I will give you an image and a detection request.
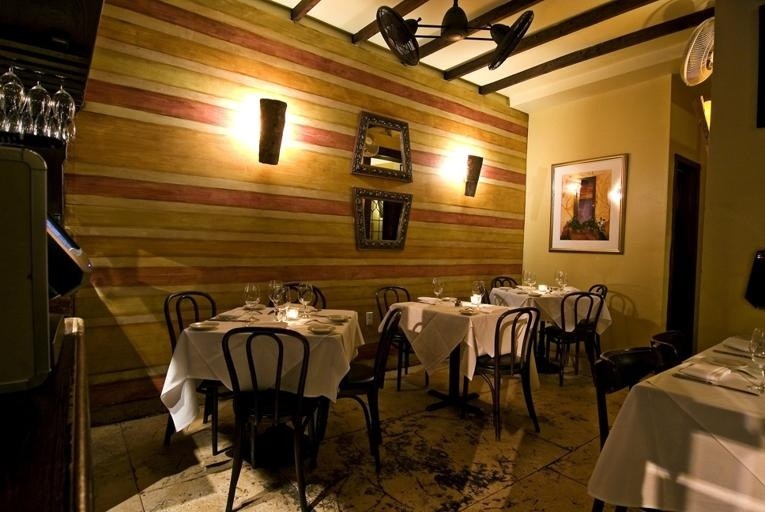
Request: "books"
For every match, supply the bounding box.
[672,360,765,395]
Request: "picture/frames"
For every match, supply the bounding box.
[548,152,630,256]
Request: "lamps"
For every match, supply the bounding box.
[464,154,484,197]
[258,98,288,166]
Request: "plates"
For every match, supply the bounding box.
[243,304,265,309]
[219,314,242,319]
[309,324,334,336]
[460,312,477,316]
[328,315,350,323]
[190,322,220,330]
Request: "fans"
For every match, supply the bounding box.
[680,16,715,86]
[376,0,534,71]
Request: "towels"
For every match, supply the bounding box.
[679,362,732,383]
[721,336,760,353]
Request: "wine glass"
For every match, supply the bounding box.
[472,280,486,309]
[523,271,527,292]
[432,277,444,300]
[268,281,280,316]
[276,287,290,321]
[559,271,567,292]
[555,271,561,287]
[750,327,765,389]
[1,68,78,139]
[298,280,313,317]
[526,272,536,292]
[242,282,260,322]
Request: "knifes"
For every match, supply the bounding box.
[672,372,759,396]
[712,349,753,359]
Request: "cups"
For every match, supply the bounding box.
[470,296,481,305]
[538,284,547,292]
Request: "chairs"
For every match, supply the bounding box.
[163,277,687,511]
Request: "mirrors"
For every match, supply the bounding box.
[351,186,413,250]
[350,110,414,183]
